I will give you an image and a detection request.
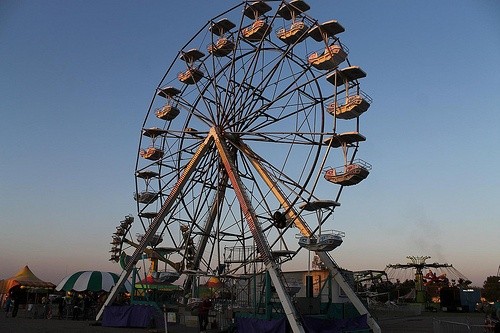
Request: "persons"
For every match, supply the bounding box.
[9,283,23,317]
[41,292,90,322]
[198,296,212,333]
[6,295,11,313]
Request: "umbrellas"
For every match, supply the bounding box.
[54,271,136,294]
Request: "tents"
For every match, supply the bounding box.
[135,271,181,304]
[0,264,57,305]
